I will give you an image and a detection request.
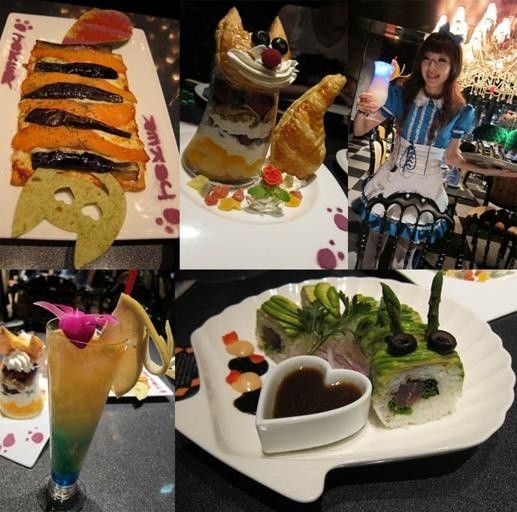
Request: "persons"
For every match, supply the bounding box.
[352,33,517,270]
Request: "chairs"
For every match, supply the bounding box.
[360,124,517,270]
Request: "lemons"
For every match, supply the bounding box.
[98,292,175,399]
[390,59,411,82]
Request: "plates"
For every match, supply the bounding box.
[179,121,348,269]
[0,12,179,241]
[0,364,174,468]
[174,270,516,503]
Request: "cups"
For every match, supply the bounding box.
[36,312,129,511]
[181,72,278,186]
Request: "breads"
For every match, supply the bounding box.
[272,72,347,178]
[257,272,465,427]
[215,6,300,95]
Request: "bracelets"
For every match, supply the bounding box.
[356,110,370,117]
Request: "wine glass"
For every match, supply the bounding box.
[362,61,393,123]
